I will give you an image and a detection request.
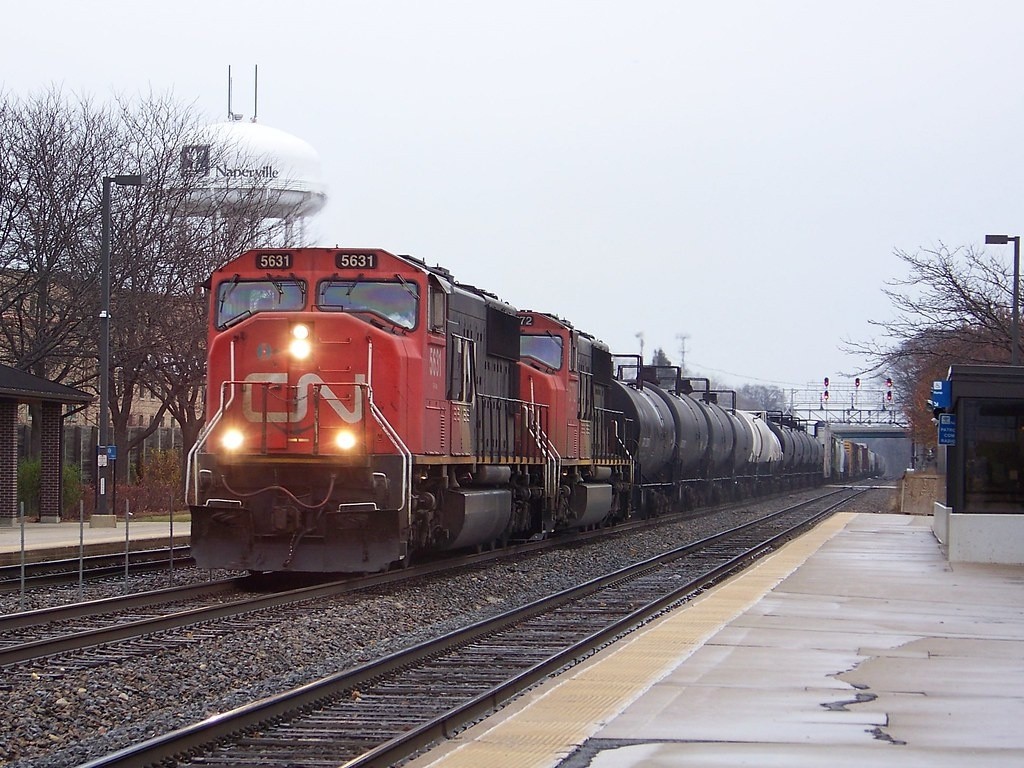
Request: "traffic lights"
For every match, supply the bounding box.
[823,377,892,401]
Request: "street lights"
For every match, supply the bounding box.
[97,175,149,514]
[986,236,1018,365]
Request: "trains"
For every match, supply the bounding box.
[181,246,887,575]
